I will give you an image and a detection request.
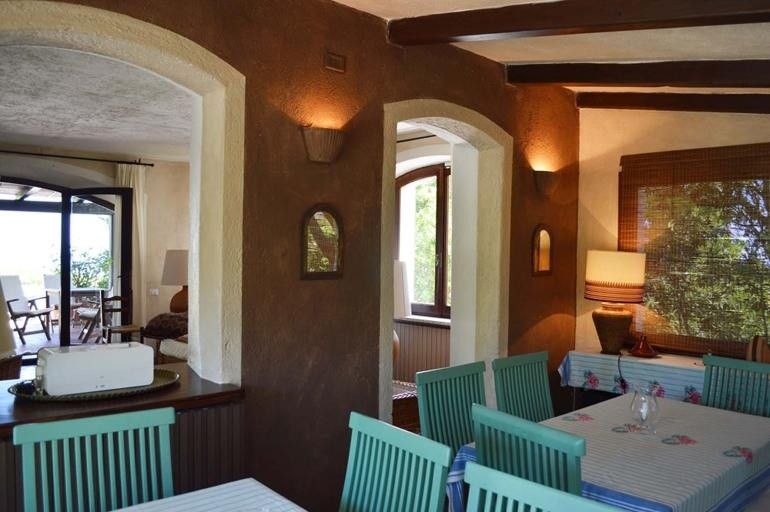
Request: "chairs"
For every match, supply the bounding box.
[100,291,145,347]
[337,411,453,512]
[1,271,114,346]
[13,406,175,512]
[417,346,770,512]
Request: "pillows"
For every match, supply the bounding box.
[146,312,191,342]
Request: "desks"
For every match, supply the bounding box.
[119,476,305,511]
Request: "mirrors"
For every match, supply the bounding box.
[302,203,346,281]
[531,223,555,278]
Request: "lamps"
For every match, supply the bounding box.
[535,169,561,197]
[303,123,349,168]
[582,248,649,358]
[161,249,189,314]
[389,258,415,373]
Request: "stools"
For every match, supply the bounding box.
[160,335,189,366]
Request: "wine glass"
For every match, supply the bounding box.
[629,382,661,436]
